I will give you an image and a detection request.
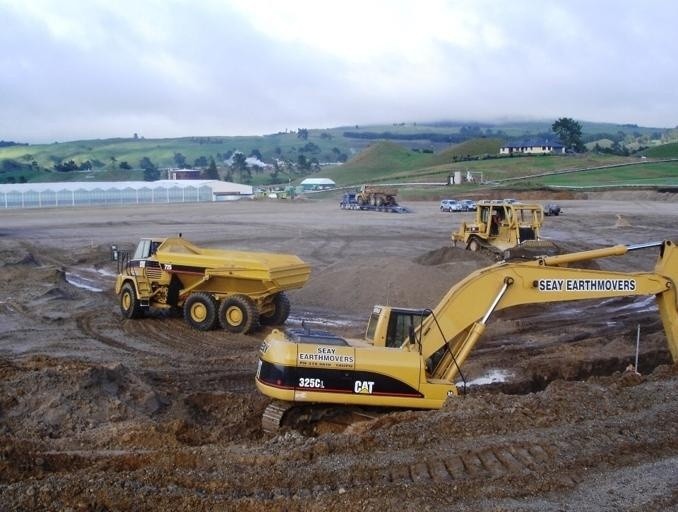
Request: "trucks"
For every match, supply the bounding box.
[107,234,316,336]
[353,183,399,207]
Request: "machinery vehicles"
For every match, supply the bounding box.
[450,203,561,262]
[249,236,678,439]
[338,191,414,214]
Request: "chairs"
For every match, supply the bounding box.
[491,216,497,235]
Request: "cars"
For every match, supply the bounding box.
[439,196,562,217]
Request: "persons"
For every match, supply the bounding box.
[492,208,503,234]
